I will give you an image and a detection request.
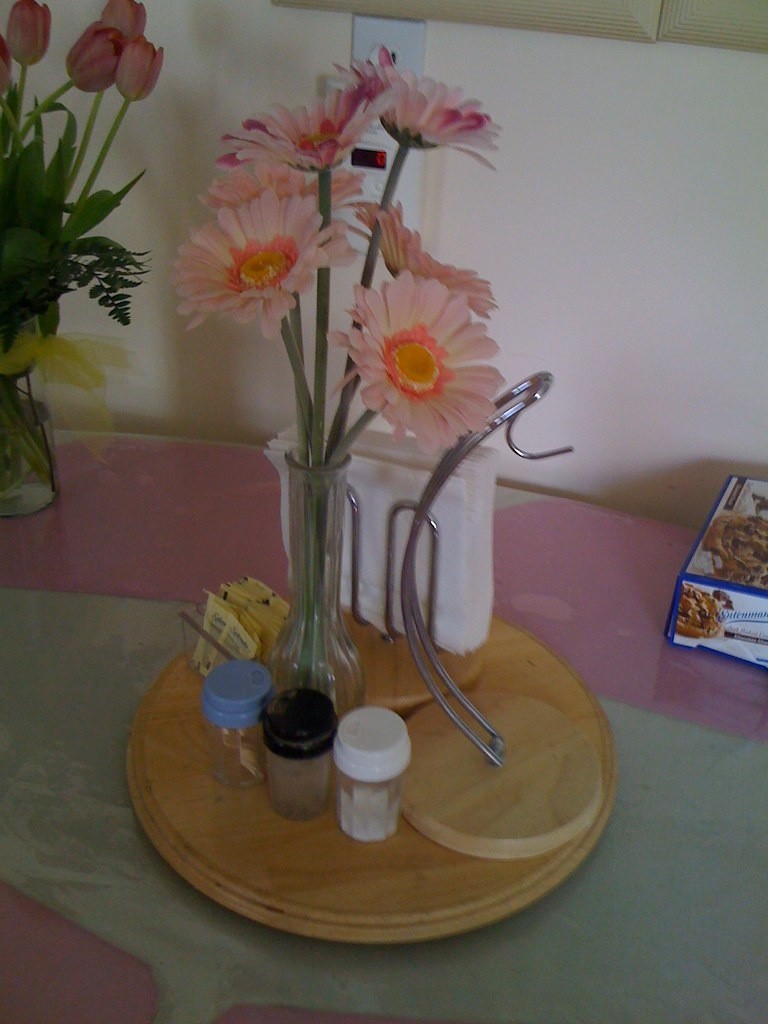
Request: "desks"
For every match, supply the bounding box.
[0,428,768,1024]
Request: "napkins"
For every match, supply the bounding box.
[264,420,498,658]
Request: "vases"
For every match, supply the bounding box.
[263,448,365,727]
[0,318,59,520]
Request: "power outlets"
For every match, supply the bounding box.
[350,13,426,79]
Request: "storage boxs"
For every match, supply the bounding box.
[661,474,767,669]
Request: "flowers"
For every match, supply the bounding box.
[172,34,505,696]
[0,0,165,487]
